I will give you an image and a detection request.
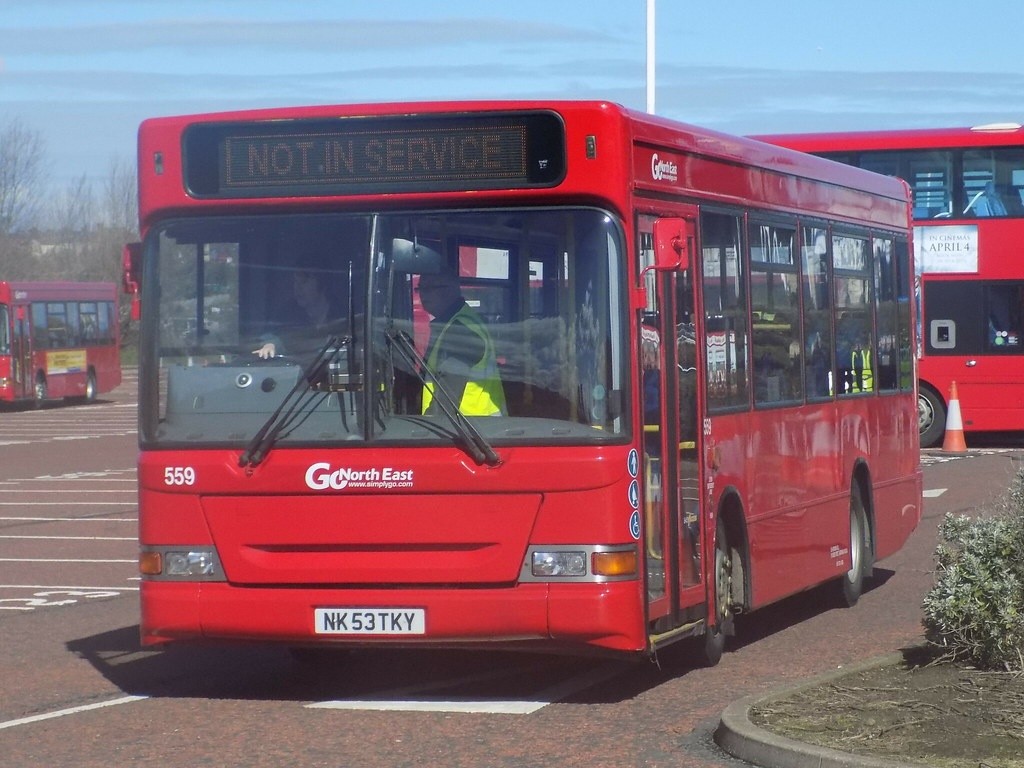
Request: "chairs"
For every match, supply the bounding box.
[944,181,1024,216]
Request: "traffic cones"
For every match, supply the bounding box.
[928,375,981,459]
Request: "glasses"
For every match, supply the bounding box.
[414,284,449,295]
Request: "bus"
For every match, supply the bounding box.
[121,100,925,671]
[410,122,1024,450]
[1,280,124,411]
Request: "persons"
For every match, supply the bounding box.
[411,263,505,420]
[848,337,874,394]
[246,257,363,363]
